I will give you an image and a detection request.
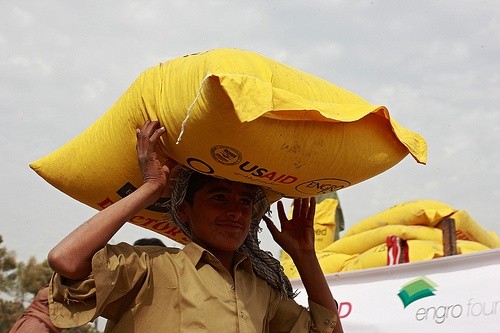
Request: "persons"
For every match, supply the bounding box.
[48,119,344,332]
[8,285,63,333]
